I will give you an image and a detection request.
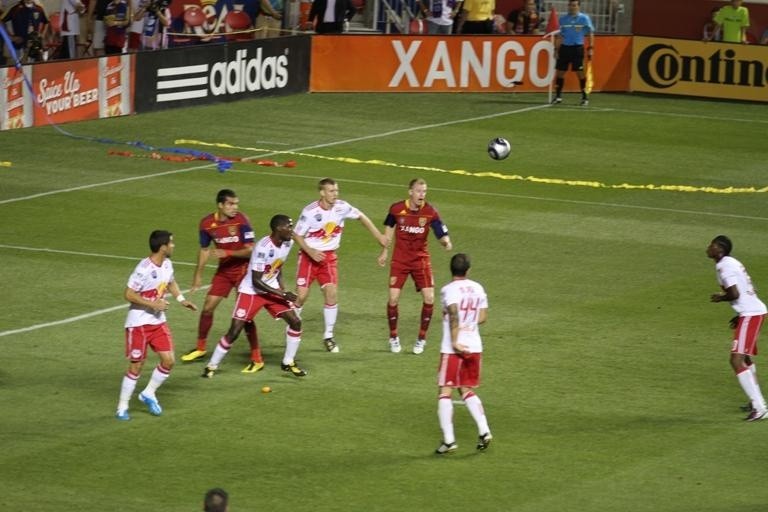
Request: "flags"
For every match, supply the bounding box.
[583,60,594,96]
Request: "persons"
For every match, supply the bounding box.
[180,189,264,373]
[552,0,596,106]
[116,230,198,421]
[286,179,392,352]
[201,214,307,377]
[377,179,452,354]
[1,0,357,70]
[379,0,547,34]
[706,236,768,422]
[204,488,228,512]
[702,0,768,45]
[436,254,493,454]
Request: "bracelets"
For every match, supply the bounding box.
[587,46,594,51]
[225,249,232,256]
[176,294,185,302]
[283,291,287,297]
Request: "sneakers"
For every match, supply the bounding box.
[551,97,561,104]
[477,433,492,451]
[580,99,589,106]
[181,336,425,380]
[138,392,162,416]
[115,409,130,421]
[739,402,763,422]
[435,439,458,455]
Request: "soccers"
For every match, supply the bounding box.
[488,138,510,160]
